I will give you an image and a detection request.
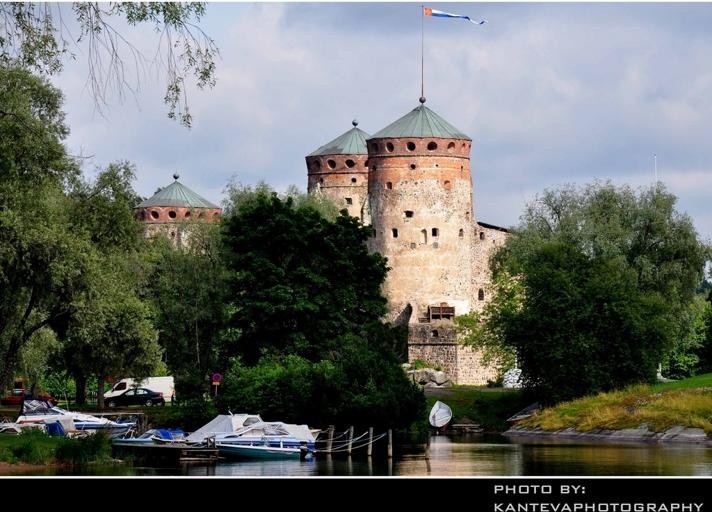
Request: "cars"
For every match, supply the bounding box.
[104,388,163,407]
[0,388,57,407]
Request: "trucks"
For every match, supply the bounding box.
[103,376,175,406]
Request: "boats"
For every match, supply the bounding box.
[506,401,540,424]
[429,399,453,428]
[0,412,322,461]
